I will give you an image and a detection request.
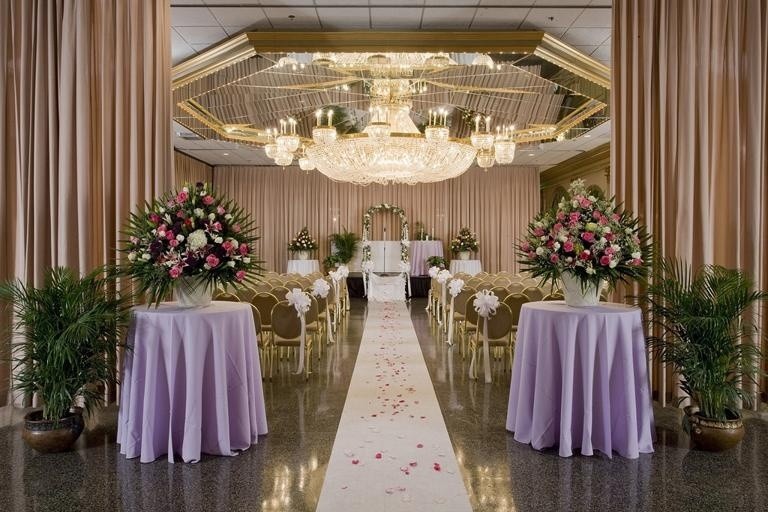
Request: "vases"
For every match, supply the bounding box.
[559,268,606,307]
[459,249,471,261]
[169,268,212,309]
[298,248,309,260]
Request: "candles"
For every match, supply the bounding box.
[419,224,436,241]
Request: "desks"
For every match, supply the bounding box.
[522,300,642,459]
[288,259,319,276]
[130,301,250,462]
[450,260,481,276]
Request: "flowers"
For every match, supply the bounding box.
[111,179,268,310]
[450,226,480,254]
[287,226,318,250]
[511,175,657,297]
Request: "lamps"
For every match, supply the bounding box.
[268,50,497,101]
[260,105,519,189]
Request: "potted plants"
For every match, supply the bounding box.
[0,264,142,454]
[623,254,768,452]
[322,225,359,274]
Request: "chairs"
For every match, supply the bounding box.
[423,266,563,383]
[211,271,349,384]
